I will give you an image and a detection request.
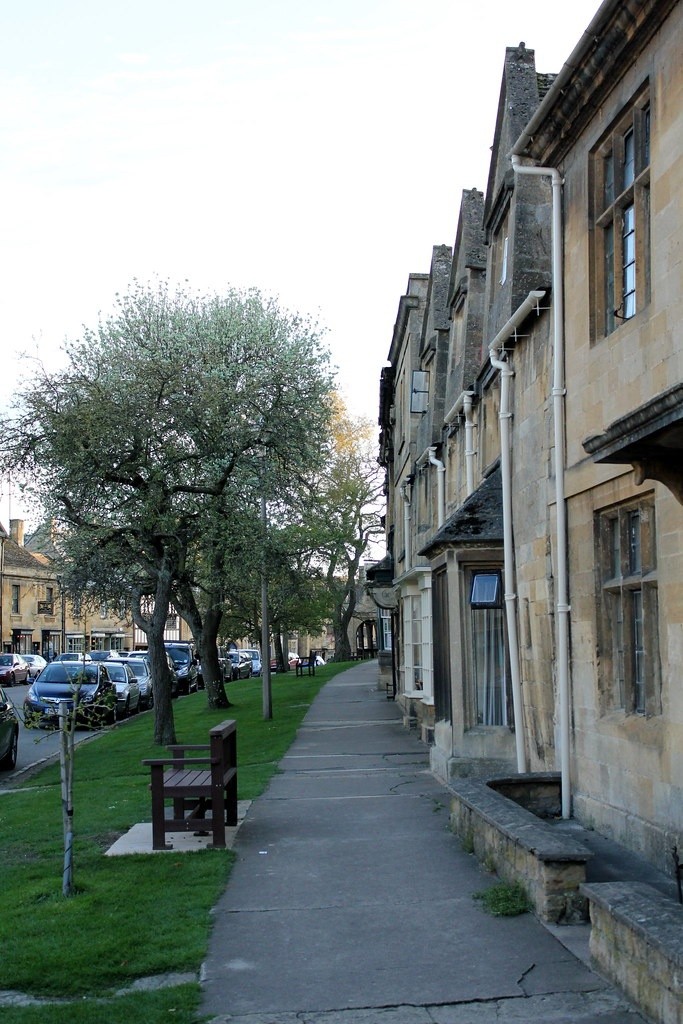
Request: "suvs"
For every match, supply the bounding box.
[87,649,122,660]
[161,643,199,695]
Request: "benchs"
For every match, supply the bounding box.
[350,650,364,661]
[140,719,237,851]
[295,650,316,677]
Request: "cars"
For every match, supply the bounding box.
[269,652,301,671]
[23,662,118,724]
[127,650,150,661]
[54,652,92,662]
[0,685,20,772]
[108,657,154,710]
[225,647,262,680]
[194,646,233,688]
[302,656,328,669]
[116,651,131,659]
[99,661,142,717]
[0,653,31,688]
[19,654,48,680]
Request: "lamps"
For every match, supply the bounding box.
[469,571,505,610]
